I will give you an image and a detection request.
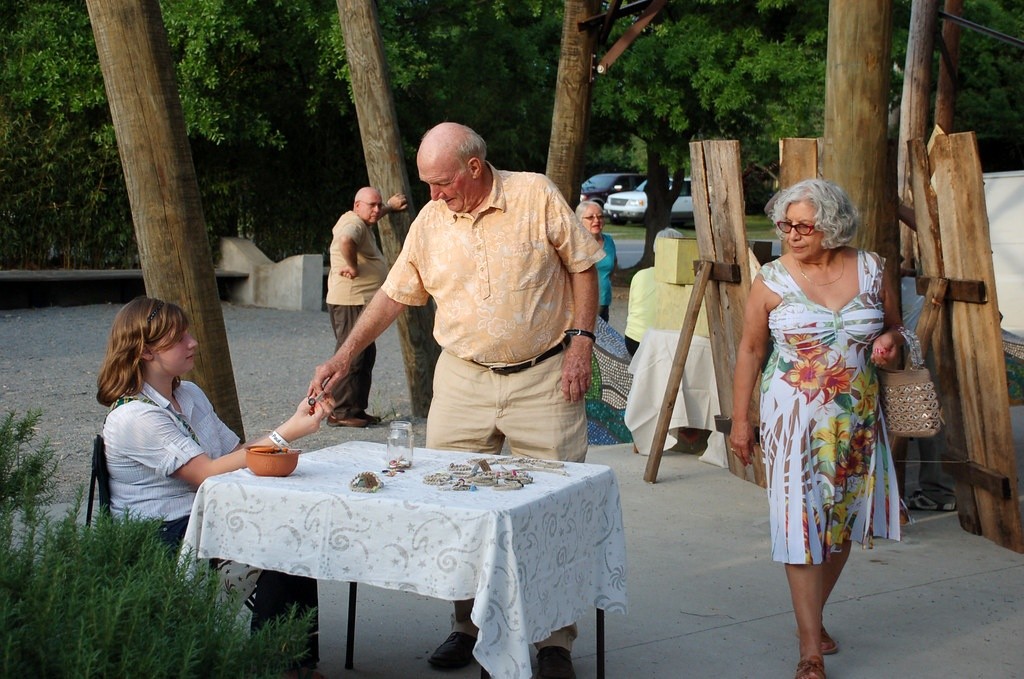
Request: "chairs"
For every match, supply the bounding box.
[84,431,306,634]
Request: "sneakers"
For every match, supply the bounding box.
[904,488,956,511]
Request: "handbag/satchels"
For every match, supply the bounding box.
[878,326,946,438]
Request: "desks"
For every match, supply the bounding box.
[176,440,627,679]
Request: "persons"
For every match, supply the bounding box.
[575,201,684,356]
[97,296,337,679]
[326,187,409,426]
[730,177,908,679]
[307,122,605,679]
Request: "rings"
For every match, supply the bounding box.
[731,447,734,451]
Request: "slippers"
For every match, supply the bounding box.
[796,624,838,679]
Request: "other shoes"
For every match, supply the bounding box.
[283,669,327,679]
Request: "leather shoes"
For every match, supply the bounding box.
[326,412,381,428]
[536,646,575,679]
[427,632,477,670]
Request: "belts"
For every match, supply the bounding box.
[470,335,571,376]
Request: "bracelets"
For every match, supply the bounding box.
[268,430,288,447]
[564,329,596,341]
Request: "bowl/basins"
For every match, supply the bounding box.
[243,445,302,477]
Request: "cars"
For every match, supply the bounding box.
[580,173,647,210]
[603,178,693,225]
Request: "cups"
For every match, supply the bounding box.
[387,421,414,469]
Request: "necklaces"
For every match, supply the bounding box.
[798,248,845,287]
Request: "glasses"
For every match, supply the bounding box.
[777,221,814,236]
[360,200,385,208]
[583,215,605,220]
